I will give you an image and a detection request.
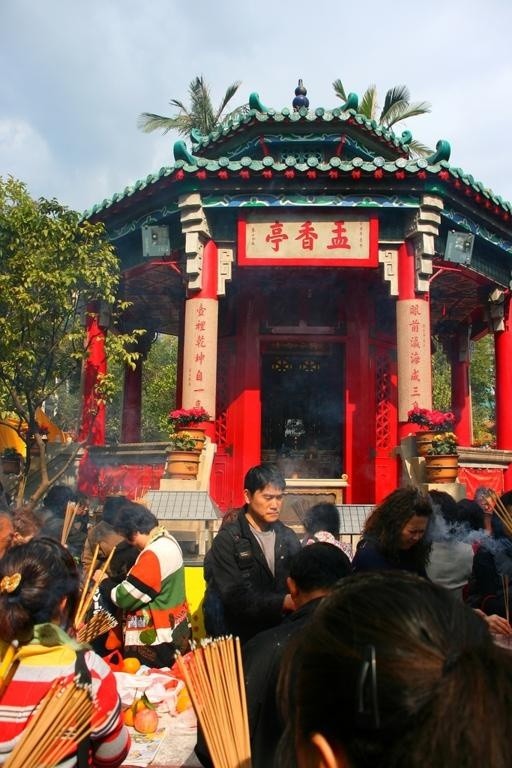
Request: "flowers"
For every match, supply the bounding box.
[169,407,210,425]
[428,432,457,455]
[407,407,455,432]
[168,429,195,451]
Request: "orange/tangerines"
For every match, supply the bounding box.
[123,658,140,674]
[135,701,147,713]
[125,709,134,726]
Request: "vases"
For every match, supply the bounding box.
[166,449,202,480]
[170,428,205,450]
[424,456,460,483]
[416,432,435,456]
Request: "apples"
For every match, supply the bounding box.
[134,709,158,733]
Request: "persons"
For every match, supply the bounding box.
[102,496,145,585]
[196,543,352,767]
[93,502,192,668]
[424,490,474,602]
[474,491,511,620]
[81,521,127,592]
[299,502,359,561]
[0,535,130,768]
[31,486,87,574]
[0,509,17,560]
[353,486,512,638]
[9,513,43,547]
[276,568,512,768]
[458,498,493,619]
[211,463,302,646]
